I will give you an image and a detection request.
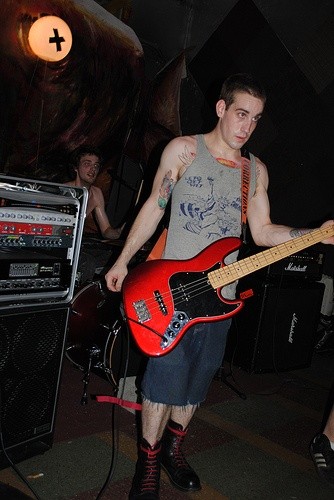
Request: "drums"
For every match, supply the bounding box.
[64,280,149,389]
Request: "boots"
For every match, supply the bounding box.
[128,437,163,500]
[158,418,202,492]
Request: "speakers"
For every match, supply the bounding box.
[0,306,70,470]
[225,275,325,375]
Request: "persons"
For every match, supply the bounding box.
[310,401,334,489]
[105,74,334,500]
[60,145,122,276]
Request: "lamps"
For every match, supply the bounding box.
[29,16,74,62]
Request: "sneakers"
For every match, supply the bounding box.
[311,434,334,486]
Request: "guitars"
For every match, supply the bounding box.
[121,224,334,358]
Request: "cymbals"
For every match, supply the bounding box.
[106,238,155,252]
